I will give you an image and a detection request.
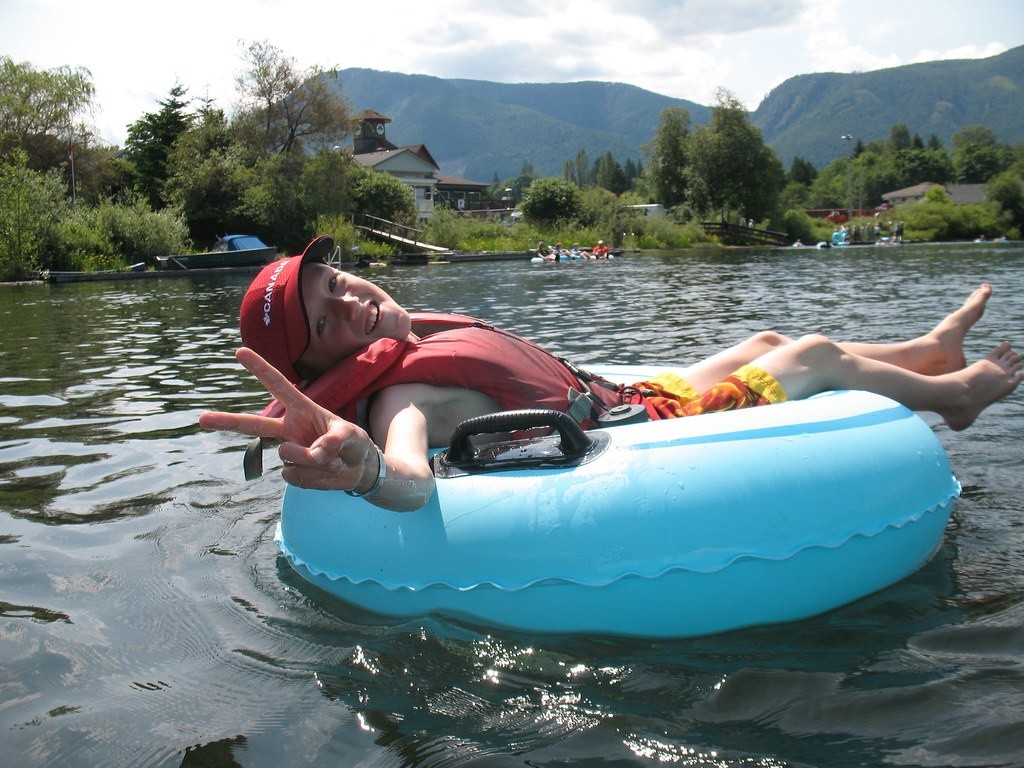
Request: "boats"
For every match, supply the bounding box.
[355,258,388,267]
[154,233,278,268]
[39,263,146,282]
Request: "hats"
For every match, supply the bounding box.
[749,219,754,222]
[598,240,603,244]
[240,234,334,388]
[556,243,562,245]
[573,243,578,245]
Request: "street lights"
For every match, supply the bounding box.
[842,134,855,217]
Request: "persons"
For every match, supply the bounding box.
[570,243,590,259]
[535,242,560,261]
[198,234,1024,513]
[748,219,753,228]
[796,239,801,247]
[836,222,904,243]
[593,240,609,260]
[554,243,573,260]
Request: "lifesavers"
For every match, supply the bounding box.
[277,364,962,640]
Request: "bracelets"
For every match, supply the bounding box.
[344,445,386,498]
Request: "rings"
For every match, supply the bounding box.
[279,442,293,463]
